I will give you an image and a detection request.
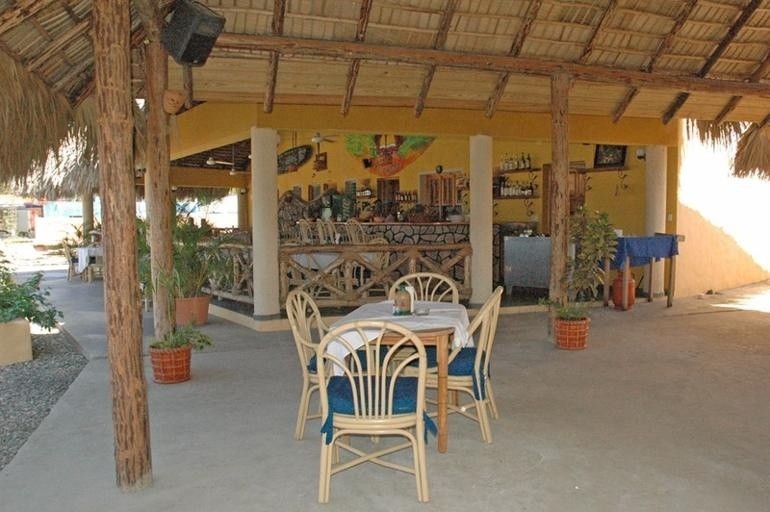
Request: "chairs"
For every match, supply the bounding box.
[279,218,390,299]
[60,240,104,283]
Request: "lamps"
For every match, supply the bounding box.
[206,143,237,176]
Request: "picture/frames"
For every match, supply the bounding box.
[593,143,627,168]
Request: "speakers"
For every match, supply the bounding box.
[364,159,369,168]
[164,0,227,68]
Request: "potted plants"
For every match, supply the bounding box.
[140,257,215,385]
[0,249,64,367]
[538,205,619,350]
[153,214,249,327]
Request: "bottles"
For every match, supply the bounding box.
[392,190,417,201]
[393,285,411,314]
[494,152,533,200]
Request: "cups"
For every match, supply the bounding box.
[405,287,417,313]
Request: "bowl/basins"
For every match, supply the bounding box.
[415,307,431,314]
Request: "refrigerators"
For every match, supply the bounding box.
[321,193,354,222]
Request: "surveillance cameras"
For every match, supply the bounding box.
[635,148,645,160]
[240,188,246,195]
[171,186,177,192]
[135,167,146,179]
